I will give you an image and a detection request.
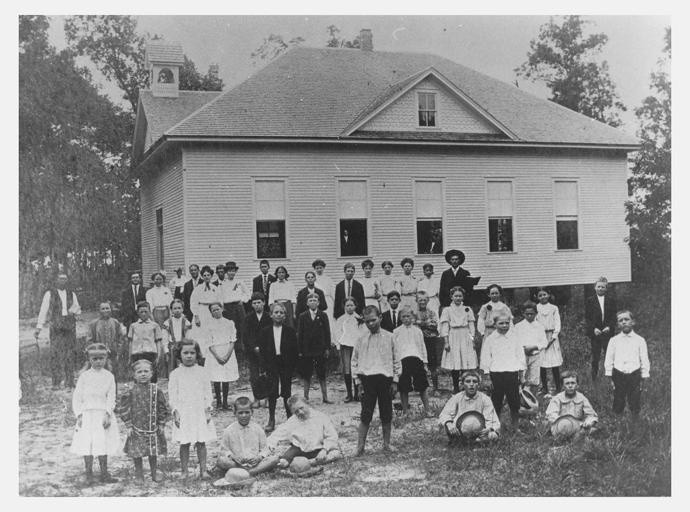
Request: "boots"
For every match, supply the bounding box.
[354,378,360,401]
[344,374,353,403]
[83,448,210,487]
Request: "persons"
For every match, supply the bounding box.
[34,273,81,391]
[604,311,651,417]
[585,278,617,381]
[545,369,598,446]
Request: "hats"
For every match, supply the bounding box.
[223,262,238,270]
[518,386,539,410]
[212,468,256,487]
[444,249,466,264]
[456,410,486,440]
[550,414,581,440]
[250,359,278,399]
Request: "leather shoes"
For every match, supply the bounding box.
[264,420,275,433]
[223,405,233,411]
[52,384,60,390]
[351,445,364,458]
[265,399,269,407]
[383,441,399,452]
[252,400,260,408]
[216,403,223,412]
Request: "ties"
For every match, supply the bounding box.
[134,285,137,304]
[263,274,267,294]
[393,310,396,326]
[348,281,350,296]
